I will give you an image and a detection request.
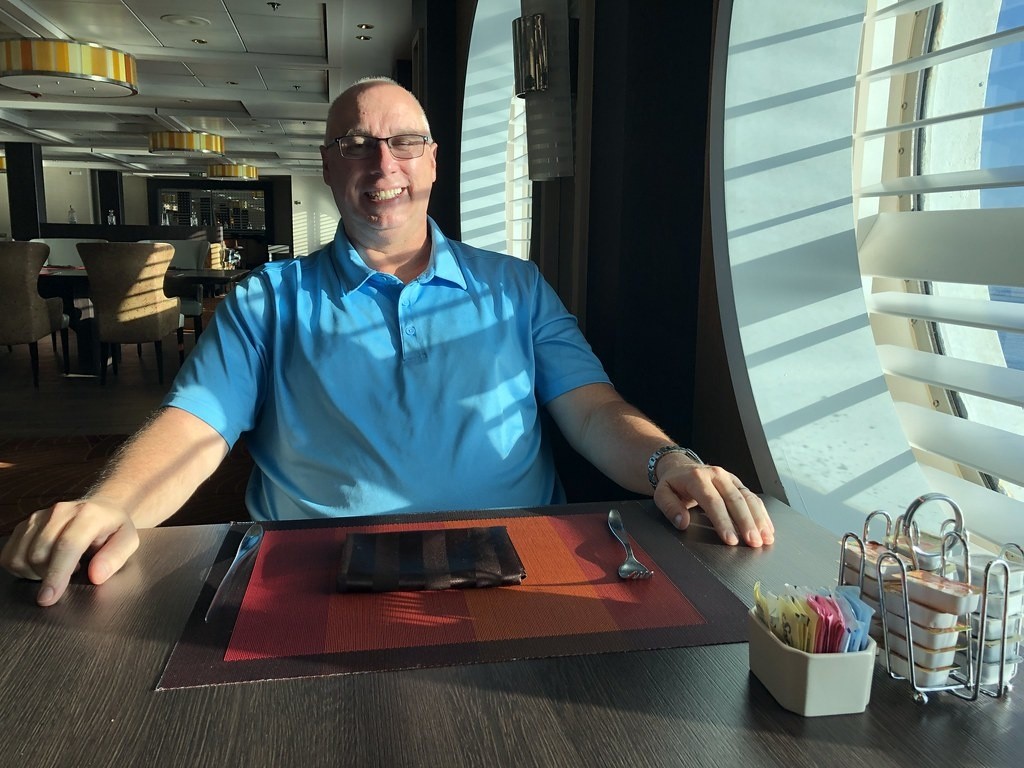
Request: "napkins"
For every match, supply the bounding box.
[341,526,526,593]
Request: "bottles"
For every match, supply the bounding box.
[161,208,170,226]
[260,224,266,230]
[108,210,116,225]
[190,212,198,226]
[224,221,228,229]
[68,208,77,224]
[247,221,252,230]
[203,220,207,225]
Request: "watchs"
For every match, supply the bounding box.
[648,446,704,490]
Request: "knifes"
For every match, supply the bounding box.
[202,523,264,625]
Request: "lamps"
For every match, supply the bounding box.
[220,200,247,209]
[512,0,574,182]
[207,165,258,181]
[0,38,138,98]
[148,131,226,158]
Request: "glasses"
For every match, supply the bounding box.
[324,132,433,160]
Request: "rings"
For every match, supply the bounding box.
[738,487,749,491]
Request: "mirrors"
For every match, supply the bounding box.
[146,178,275,240]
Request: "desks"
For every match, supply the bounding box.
[0,492,1024,768]
[38,267,252,371]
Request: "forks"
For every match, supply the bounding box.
[608,507,654,581]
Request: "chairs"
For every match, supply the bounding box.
[0,239,211,390]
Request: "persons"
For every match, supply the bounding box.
[1,78,776,608]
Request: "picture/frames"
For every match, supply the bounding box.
[411,28,423,106]
